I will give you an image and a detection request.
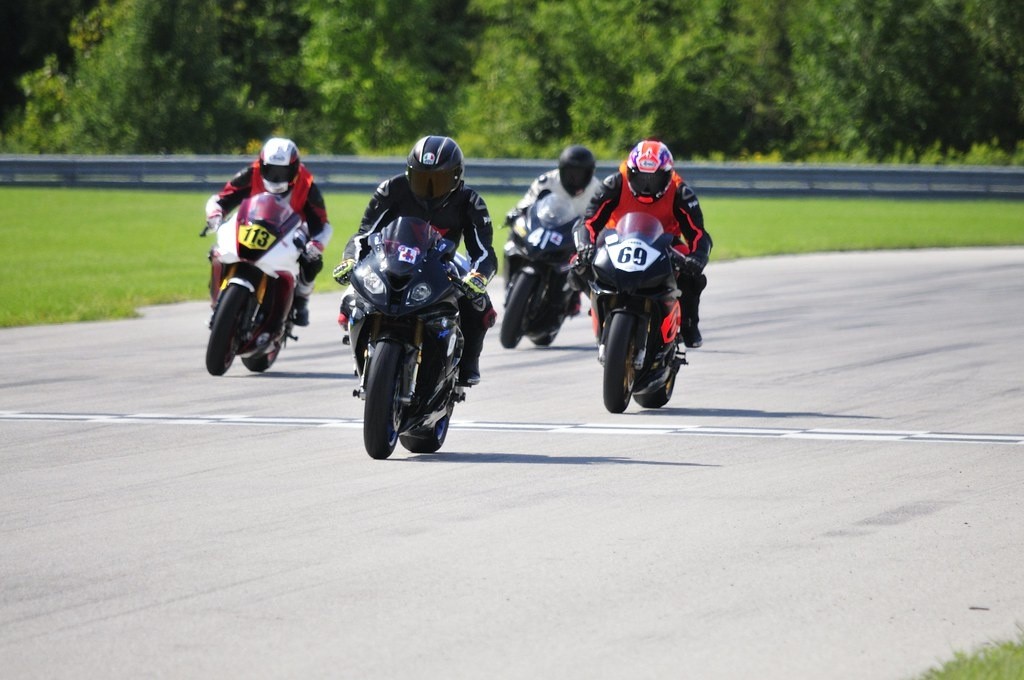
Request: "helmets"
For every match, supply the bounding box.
[627,140,674,204]
[260,137,299,196]
[407,135,464,211]
[558,146,595,197]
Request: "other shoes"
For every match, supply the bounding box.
[288,295,308,326]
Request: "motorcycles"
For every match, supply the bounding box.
[341,215,479,460]
[575,209,688,414]
[200,190,322,377]
[499,190,585,349]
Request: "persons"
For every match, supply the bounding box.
[501,147,602,315]
[205,138,333,325]
[570,140,713,348]
[338,136,498,383]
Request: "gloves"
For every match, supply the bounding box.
[305,240,325,260]
[507,209,522,226]
[681,252,709,278]
[207,209,223,233]
[333,259,355,287]
[462,272,487,299]
[576,242,595,263]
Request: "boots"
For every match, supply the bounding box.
[457,295,497,385]
[677,274,707,348]
[338,294,356,345]
